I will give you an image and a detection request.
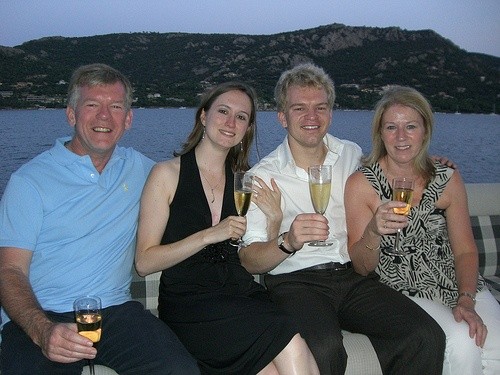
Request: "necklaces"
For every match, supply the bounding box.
[199,166,223,205]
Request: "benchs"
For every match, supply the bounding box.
[82,183,500,375]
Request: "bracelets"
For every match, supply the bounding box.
[361,232,380,251]
[458,291,476,305]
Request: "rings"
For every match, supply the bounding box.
[384,221,387,228]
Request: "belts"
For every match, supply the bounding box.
[306,261,354,271]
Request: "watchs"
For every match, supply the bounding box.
[277,232,297,258]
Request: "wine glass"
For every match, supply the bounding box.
[228,169,255,247]
[384,178,415,256]
[72,295,103,375]
[308,164,334,247]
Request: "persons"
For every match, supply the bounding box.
[241,62,460,375]
[0,63,200,375]
[345,85,500,375]
[136,81,321,375]
